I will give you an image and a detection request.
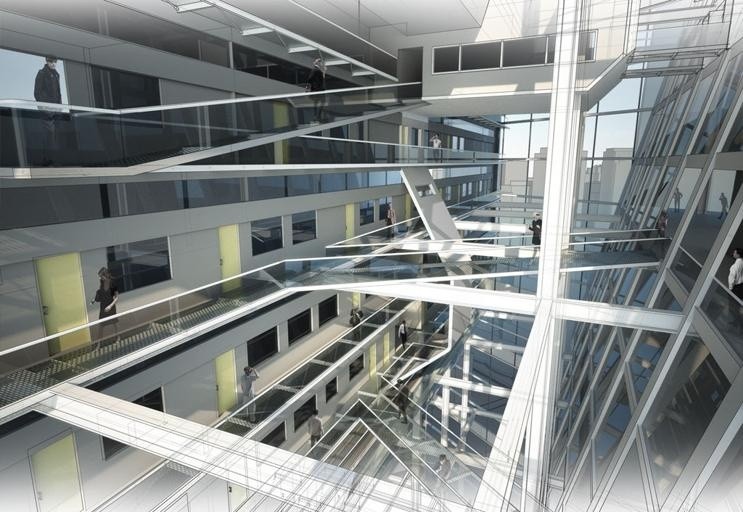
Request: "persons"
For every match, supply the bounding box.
[90,267,121,353]
[717,193,729,220]
[306,58,326,121]
[727,247,743,327]
[385,202,397,238]
[34,54,61,167]
[398,320,409,351]
[428,133,442,163]
[239,366,259,423]
[672,188,684,213]
[309,378,450,491]
[527,213,542,266]
[349,303,364,340]
